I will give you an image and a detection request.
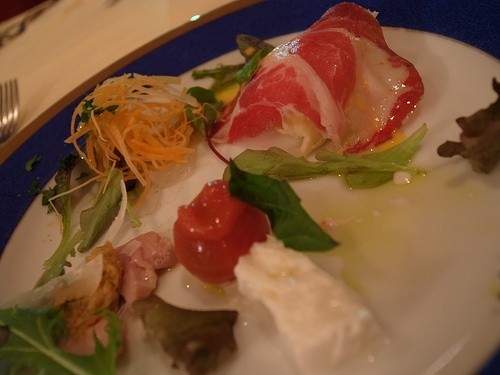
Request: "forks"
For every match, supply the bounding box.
[0,78,20,142]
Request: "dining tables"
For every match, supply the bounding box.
[1,0,500,375]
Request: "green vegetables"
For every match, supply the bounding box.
[181,36,427,252]
[0,152,144,375]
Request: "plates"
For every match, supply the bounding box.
[0,2,499,374]
